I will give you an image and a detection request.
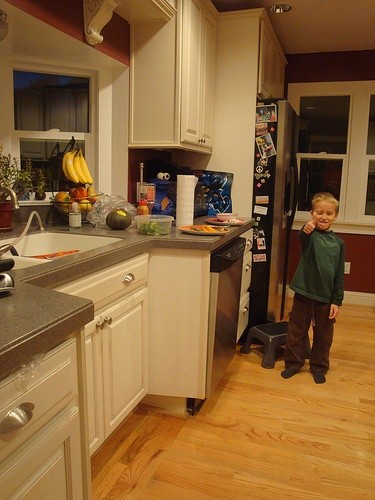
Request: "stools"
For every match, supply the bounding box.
[240,322,312,369]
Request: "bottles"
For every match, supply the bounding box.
[69,202,81,227]
[136,200,149,215]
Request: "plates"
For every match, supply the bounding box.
[205,219,243,225]
[179,226,230,234]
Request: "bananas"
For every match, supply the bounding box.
[62,146,93,184]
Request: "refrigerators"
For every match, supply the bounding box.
[250,99,300,327]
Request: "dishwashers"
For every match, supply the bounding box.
[145,236,246,412]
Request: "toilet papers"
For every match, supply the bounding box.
[176,176,198,227]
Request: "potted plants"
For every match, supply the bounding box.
[0,144,46,231]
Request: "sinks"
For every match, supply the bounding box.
[0,231,124,261]
[0,253,47,271]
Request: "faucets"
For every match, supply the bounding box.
[0,186,20,209]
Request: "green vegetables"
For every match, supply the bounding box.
[140,221,168,235]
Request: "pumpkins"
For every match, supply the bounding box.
[106,209,132,230]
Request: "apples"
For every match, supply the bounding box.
[55,186,99,214]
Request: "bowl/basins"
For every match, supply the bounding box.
[135,215,174,235]
[217,213,238,221]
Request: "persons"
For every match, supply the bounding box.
[281,192,345,384]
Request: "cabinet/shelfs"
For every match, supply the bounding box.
[129,0,216,154]
[237,228,253,342]
[51,251,149,456]
[256,22,285,100]
[0,326,92,500]
